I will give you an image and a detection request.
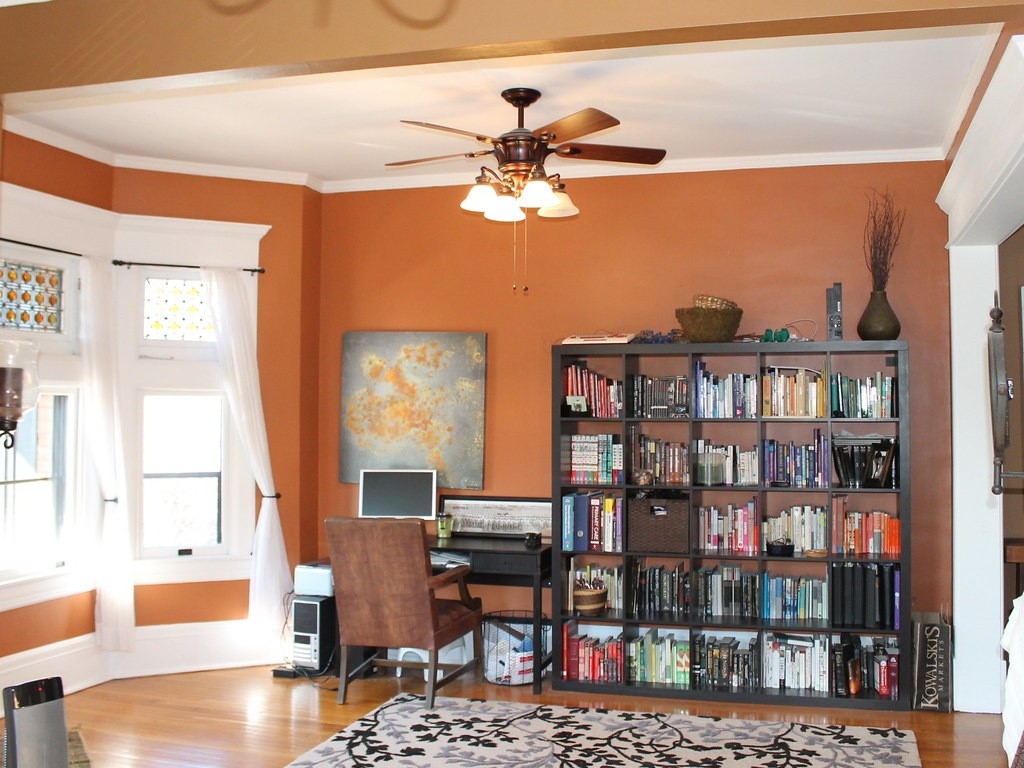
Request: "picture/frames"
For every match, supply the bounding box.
[439,494,554,539]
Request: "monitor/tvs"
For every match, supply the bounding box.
[359,470,436,521]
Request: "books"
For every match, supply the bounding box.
[560,491,621,554]
[562,620,900,703]
[566,556,901,631]
[699,495,901,556]
[561,426,900,490]
[563,361,897,418]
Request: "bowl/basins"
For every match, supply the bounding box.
[574,589,607,616]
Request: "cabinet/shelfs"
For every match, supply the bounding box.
[550,342,911,710]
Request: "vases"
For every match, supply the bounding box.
[857,291,901,340]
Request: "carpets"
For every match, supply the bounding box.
[284,691,918,768]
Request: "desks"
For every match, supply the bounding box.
[334,534,552,695]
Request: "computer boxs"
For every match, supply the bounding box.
[292,595,337,672]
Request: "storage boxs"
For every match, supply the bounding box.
[482,609,549,686]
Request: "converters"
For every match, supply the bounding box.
[272,668,296,679]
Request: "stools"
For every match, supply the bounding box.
[396,636,468,683]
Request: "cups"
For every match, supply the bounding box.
[437,513,453,538]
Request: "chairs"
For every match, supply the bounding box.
[325,518,483,710]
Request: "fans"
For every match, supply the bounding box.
[384,87,667,168]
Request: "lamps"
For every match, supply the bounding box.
[460,165,579,291]
[0,339,42,449]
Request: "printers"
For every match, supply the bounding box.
[294,557,334,597]
